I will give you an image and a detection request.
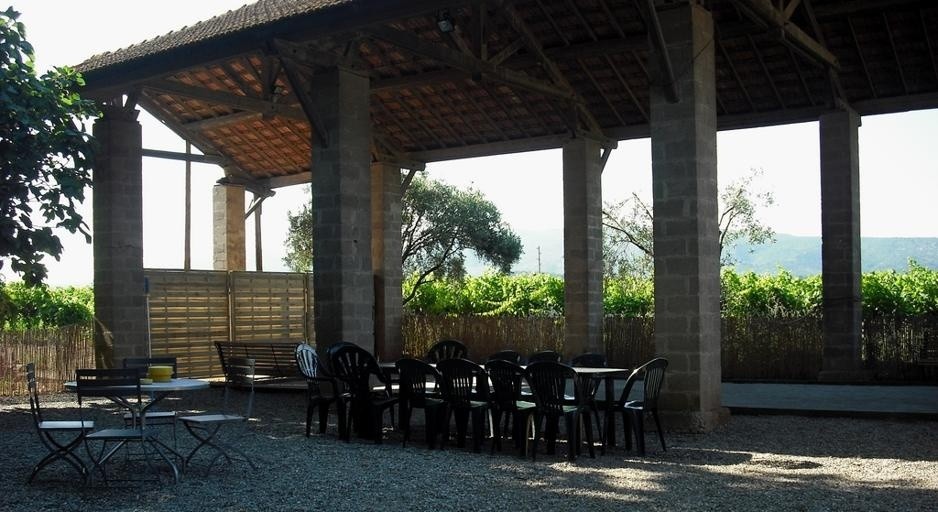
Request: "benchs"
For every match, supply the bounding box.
[214,339,306,398]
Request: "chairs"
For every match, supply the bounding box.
[26,358,260,485]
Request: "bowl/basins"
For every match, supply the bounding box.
[147,366,173,382]
